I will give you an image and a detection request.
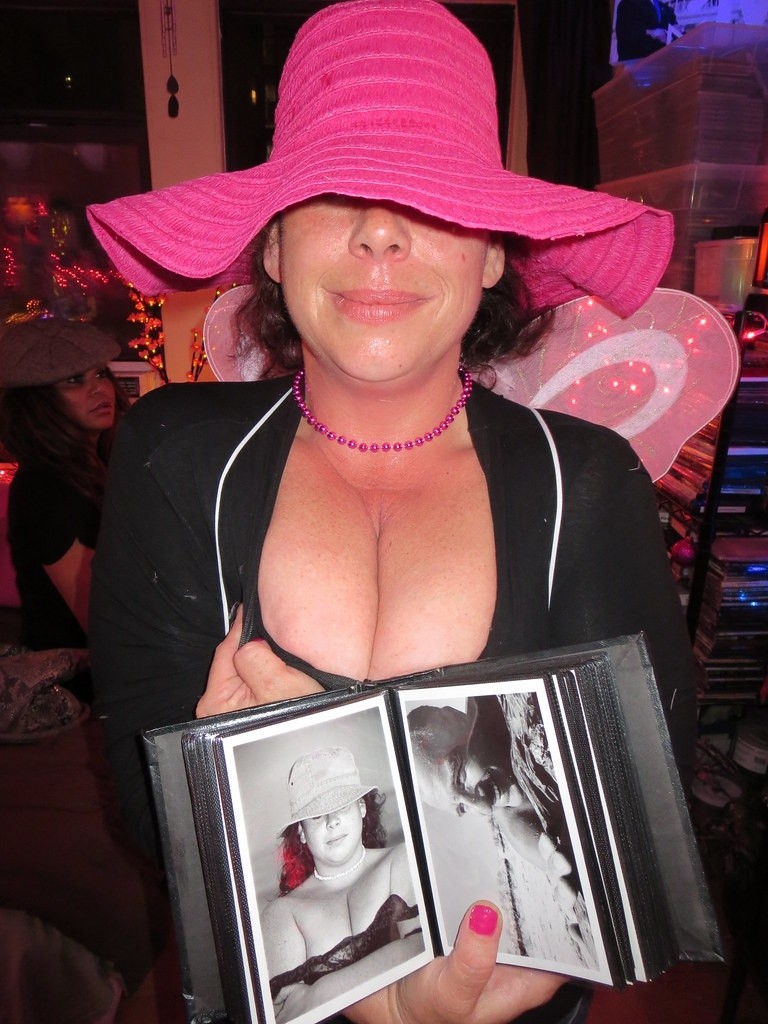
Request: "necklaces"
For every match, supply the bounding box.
[293,365,473,452]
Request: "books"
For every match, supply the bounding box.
[646,367,768,691]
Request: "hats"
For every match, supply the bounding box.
[85,0,674,322]
[274,746,376,836]
[1,316,119,390]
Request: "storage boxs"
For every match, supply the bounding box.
[593,23,768,182]
[594,163,768,293]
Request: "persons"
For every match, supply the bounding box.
[256,745,425,1024]
[403,693,587,903]
[85,0,699,1023]
[0,317,131,707]
